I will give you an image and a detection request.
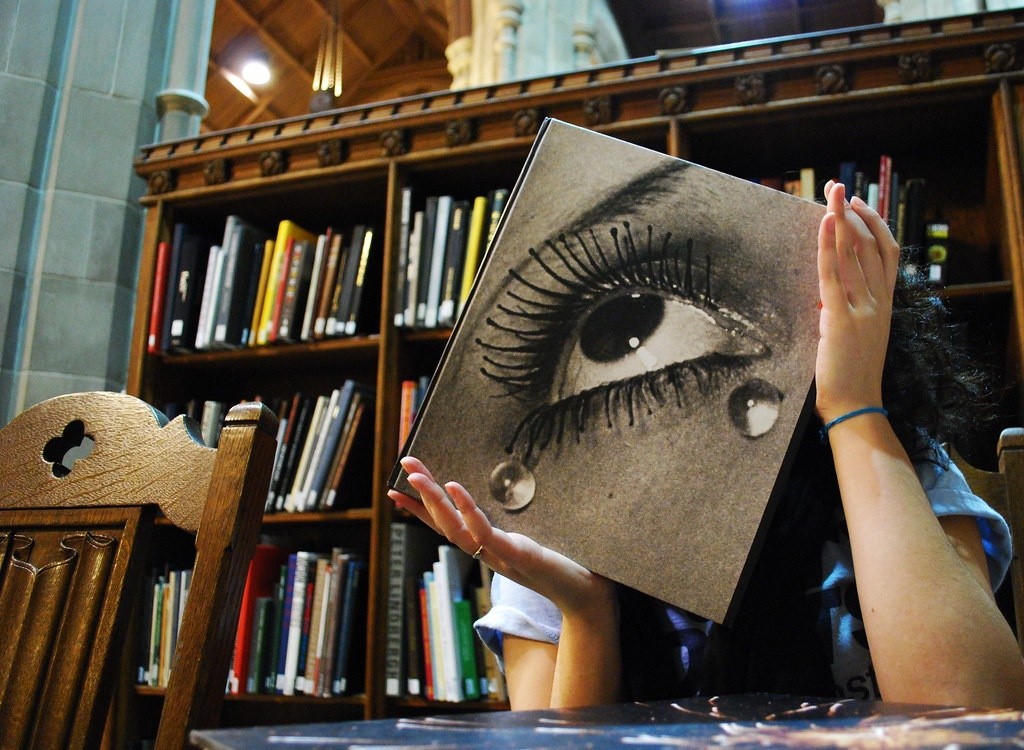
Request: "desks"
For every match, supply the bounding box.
[187,694,1023,749]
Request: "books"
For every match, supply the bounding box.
[145,152,925,353]
[133,545,506,705]
[164,375,428,517]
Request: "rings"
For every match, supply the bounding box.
[471,546,484,560]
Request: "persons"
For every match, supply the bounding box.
[388,179,1024,712]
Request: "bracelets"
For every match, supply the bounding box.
[819,407,888,439]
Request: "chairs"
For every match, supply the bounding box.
[938,426,1023,656]
[134,5,1023,726]
[0,393,280,750]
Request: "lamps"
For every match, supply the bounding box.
[238,32,270,85]
